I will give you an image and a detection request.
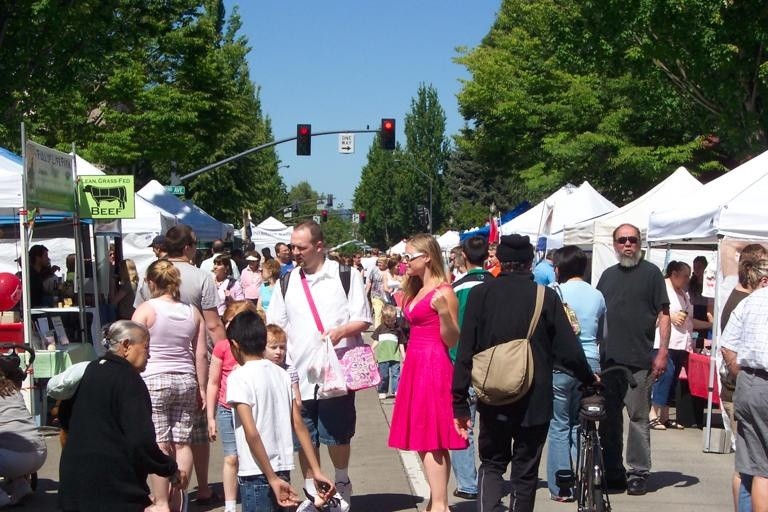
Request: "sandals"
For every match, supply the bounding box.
[664,418,685,430]
[649,416,667,431]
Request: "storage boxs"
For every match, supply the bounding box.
[0,311,2,322]
[3,310,20,323]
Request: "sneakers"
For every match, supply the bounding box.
[627,477,649,495]
[378,392,386,400]
[329,475,353,512]
[453,488,477,500]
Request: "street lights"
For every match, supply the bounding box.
[394,158,434,236]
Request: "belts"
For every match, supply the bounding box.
[740,364,768,380]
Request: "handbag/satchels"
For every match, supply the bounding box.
[334,342,383,393]
[468,337,536,407]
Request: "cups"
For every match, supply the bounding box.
[695,348,711,355]
[678,309,688,326]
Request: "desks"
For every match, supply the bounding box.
[18,341,99,430]
[688,349,721,410]
[0,321,24,359]
[30,306,116,358]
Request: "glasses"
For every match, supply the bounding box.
[401,252,424,263]
[613,236,641,245]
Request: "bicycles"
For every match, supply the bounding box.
[550,364,638,510]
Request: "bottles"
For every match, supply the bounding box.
[53,274,65,307]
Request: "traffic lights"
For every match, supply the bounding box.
[358,211,367,224]
[322,210,328,222]
[380,118,396,150]
[296,124,311,155]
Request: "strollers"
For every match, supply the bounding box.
[0,342,38,493]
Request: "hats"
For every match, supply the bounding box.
[245,255,260,261]
[145,234,166,250]
[495,232,536,263]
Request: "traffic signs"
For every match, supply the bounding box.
[165,186,185,195]
[337,132,356,154]
